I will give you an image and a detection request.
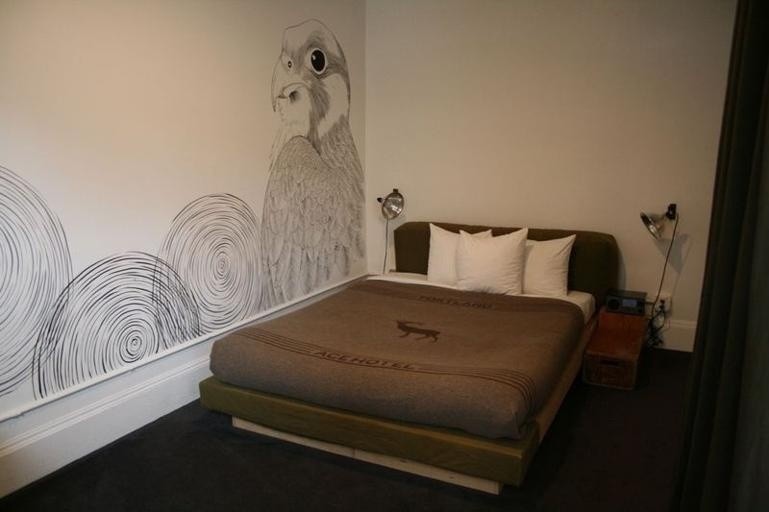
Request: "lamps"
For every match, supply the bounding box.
[377,188,405,274]
[640,203,682,347]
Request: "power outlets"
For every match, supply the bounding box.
[654,293,671,313]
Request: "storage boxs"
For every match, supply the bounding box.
[582,311,649,390]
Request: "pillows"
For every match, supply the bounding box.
[427,223,576,298]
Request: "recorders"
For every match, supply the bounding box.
[606,289,648,315]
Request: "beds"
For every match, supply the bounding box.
[199,222,618,500]
